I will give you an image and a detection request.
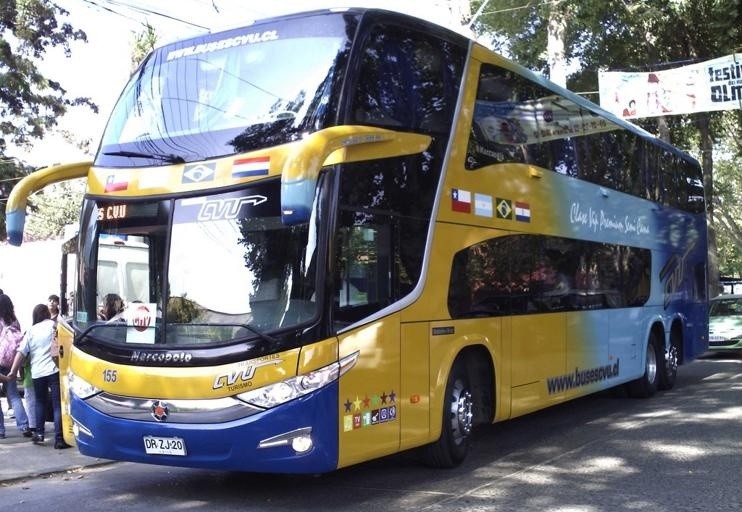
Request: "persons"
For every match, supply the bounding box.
[100,294,124,321]
[0,289,71,449]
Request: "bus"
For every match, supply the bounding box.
[5,5,708,475]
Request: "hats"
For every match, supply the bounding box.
[648,74,659,83]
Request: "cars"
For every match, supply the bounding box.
[708,294,742,350]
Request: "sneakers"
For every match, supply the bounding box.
[54,437,70,449]
[0,431,5,439]
[22,428,44,442]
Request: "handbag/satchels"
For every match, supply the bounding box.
[50,330,60,368]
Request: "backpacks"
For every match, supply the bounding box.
[0,317,24,370]
